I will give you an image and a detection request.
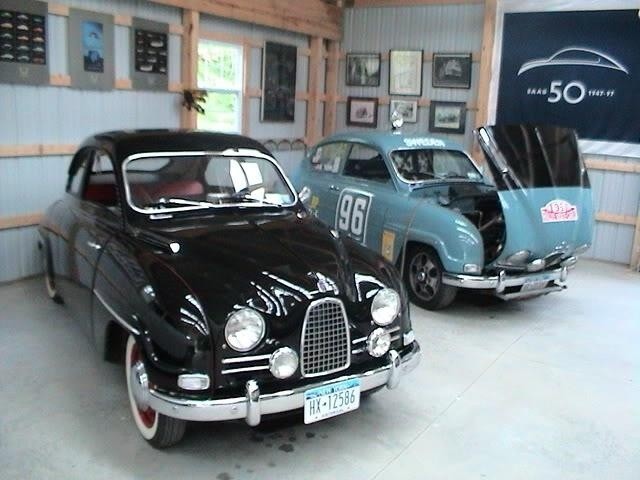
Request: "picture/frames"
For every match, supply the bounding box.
[260,39,298,123]
[345,48,473,135]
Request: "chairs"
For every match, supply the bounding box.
[354,159,387,177]
[86,174,145,204]
[154,180,204,198]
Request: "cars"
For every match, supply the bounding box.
[35,128,422,449]
[272,124,598,311]
